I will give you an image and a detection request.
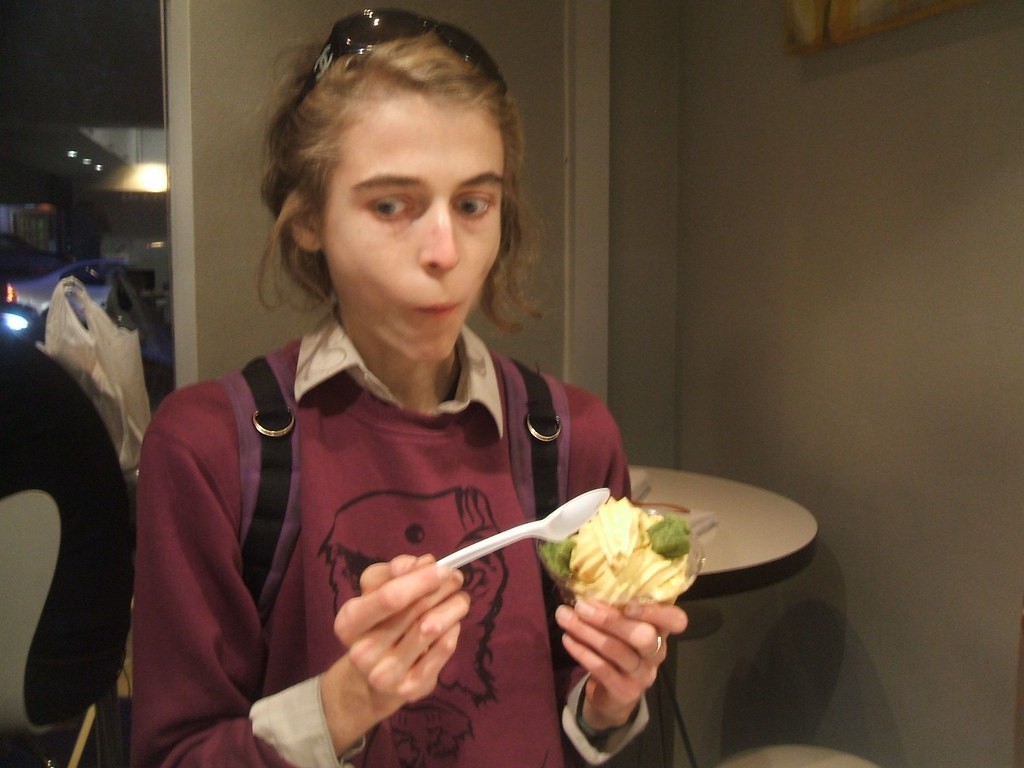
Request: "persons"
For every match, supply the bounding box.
[129,16,688,768]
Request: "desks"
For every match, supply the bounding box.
[626,465,818,767]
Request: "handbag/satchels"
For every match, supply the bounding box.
[36,276,152,474]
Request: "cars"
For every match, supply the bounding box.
[7,256,124,321]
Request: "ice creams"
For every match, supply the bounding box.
[538,495,696,606]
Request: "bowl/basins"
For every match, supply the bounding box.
[537,503,703,607]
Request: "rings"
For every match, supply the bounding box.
[640,634,662,658]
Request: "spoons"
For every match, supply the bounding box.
[436,487,612,571]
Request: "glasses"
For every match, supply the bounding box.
[296,8,508,117]
